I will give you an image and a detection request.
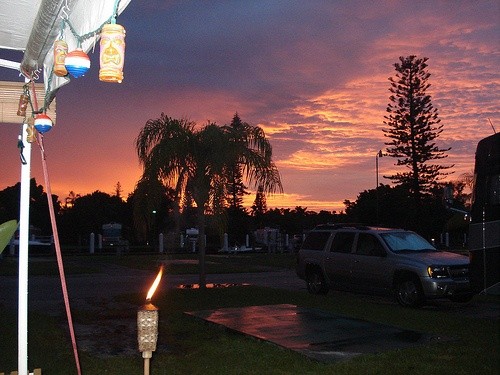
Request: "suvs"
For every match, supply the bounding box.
[102,223,123,247]
[294,221,472,311]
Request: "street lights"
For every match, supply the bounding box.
[375,149,383,187]
